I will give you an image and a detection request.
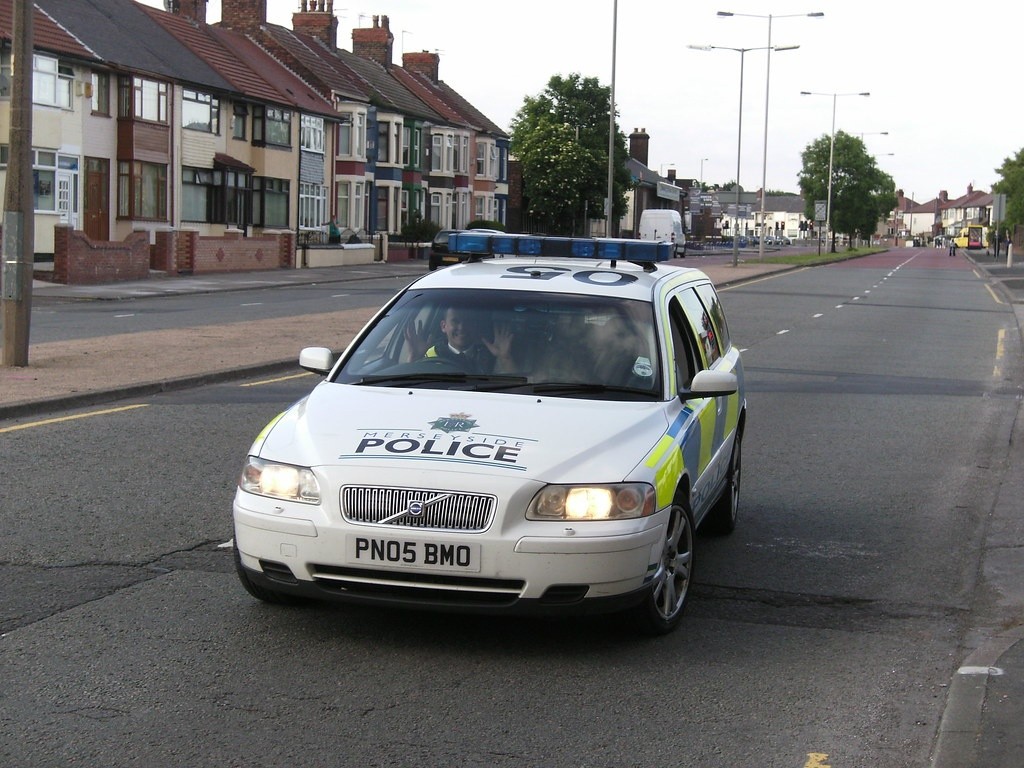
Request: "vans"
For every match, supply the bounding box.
[950,224,990,249]
[639,209,687,259]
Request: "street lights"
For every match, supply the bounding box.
[661,163,675,176]
[700,158,710,183]
[801,92,872,256]
[847,130,889,143]
[687,43,800,266]
[717,11,824,262]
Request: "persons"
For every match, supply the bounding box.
[949,238,956,256]
[991,230,1001,257]
[1004,229,1011,255]
[933,237,947,249]
[322,215,341,244]
[403,300,517,375]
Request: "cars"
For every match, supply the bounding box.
[232,230,748,639]
[429,229,505,272]
